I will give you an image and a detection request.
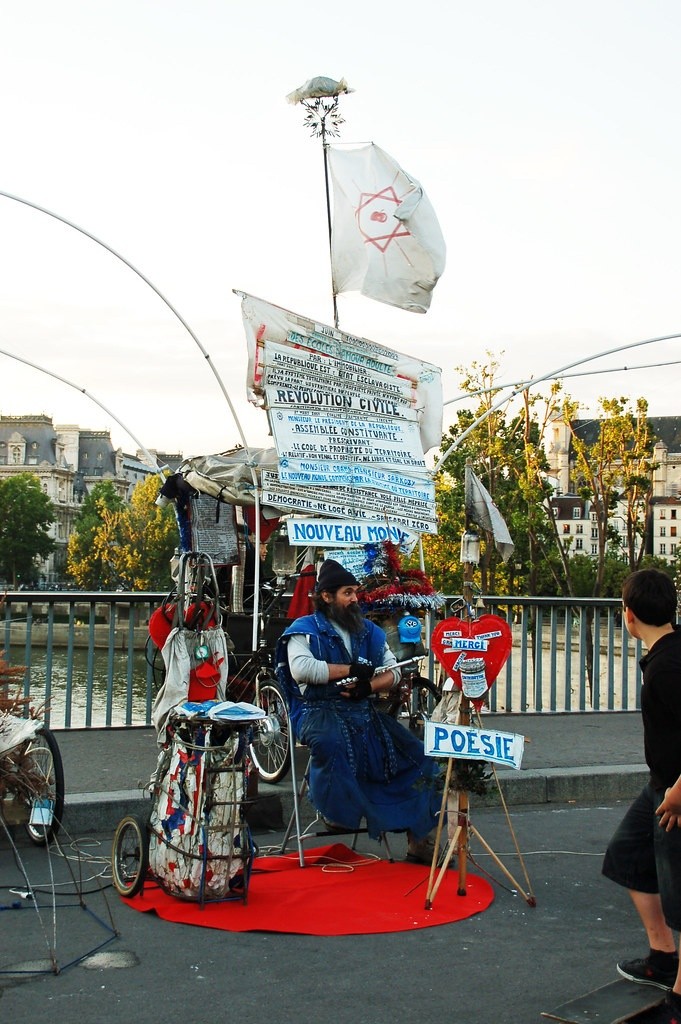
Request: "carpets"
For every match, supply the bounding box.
[121,842,497,937]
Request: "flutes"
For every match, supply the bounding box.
[335,655,426,686]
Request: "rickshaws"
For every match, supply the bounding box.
[175,444,448,785]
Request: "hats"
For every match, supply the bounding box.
[318,559,356,591]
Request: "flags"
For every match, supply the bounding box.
[465,467,514,563]
[328,144,446,314]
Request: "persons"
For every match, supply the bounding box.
[600,569,681,1024]
[201,544,277,742]
[275,559,455,868]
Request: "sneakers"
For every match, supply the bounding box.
[626,992,681,1024]
[616,958,678,991]
[320,809,351,833]
[407,835,454,866]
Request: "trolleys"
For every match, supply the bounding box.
[111,551,259,899]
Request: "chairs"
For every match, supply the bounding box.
[262,617,396,867]
